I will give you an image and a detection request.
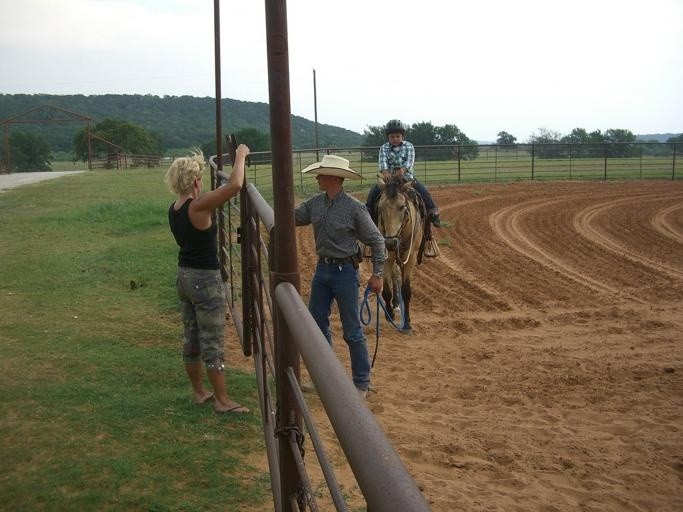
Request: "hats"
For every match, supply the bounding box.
[300,154,368,181]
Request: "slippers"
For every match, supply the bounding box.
[215,406,250,414]
[195,392,216,405]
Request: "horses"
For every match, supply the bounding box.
[371,173,427,337]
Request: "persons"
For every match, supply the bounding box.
[293,150,389,401]
[364,120,443,229]
[164,143,252,415]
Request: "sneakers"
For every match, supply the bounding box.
[431,214,441,228]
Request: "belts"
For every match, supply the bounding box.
[319,255,353,264]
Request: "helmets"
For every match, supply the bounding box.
[385,119,406,131]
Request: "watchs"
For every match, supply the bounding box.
[373,272,383,279]
